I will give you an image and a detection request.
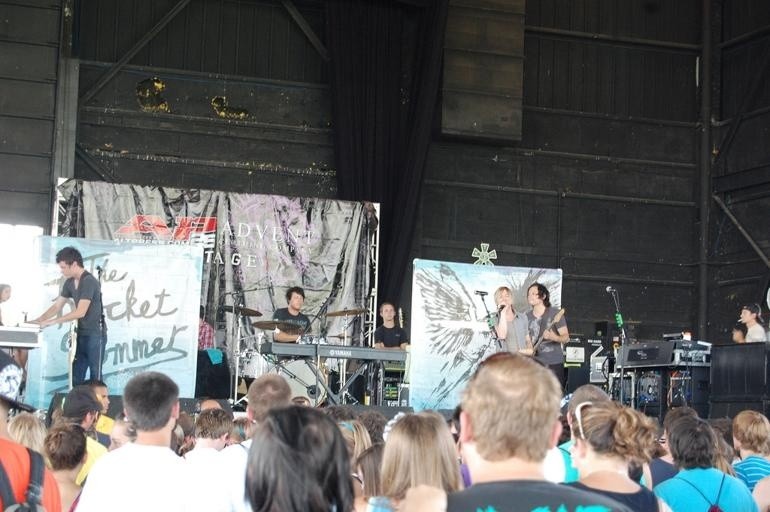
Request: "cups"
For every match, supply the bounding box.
[575,400,593,442]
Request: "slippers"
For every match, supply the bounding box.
[672,472,730,512]
[0,435,47,512]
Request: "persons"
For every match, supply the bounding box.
[732,319,746,341]
[1,380,137,510]
[197,305,215,350]
[321,405,472,510]
[523,283,570,393]
[373,303,408,350]
[680,330,693,341]
[732,411,765,493]
[76,372,202,511]
[1,284,28,381]
[447,353,632,511]
[651,416,759,510]
[272,286,312,363]
[26,247,107,389]
[741,304,767,342]
[544,385,673,511]
[642,407,698,492]
[489,287,533,355]
[171,374,354,510]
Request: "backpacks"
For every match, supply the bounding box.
[269,359,327,409]
[241,348,277,379]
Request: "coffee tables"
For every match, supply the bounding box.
[253,321,300,332]
[325,309,367,317]
[222,305,263,317]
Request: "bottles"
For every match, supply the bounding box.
[0,349,37,415]
[741,302,765,324]
[61,385,103,419]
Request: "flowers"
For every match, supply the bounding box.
[271,282,280,295]
[605,286,621,294]
[474,289,489,297]
[494,304,506,317]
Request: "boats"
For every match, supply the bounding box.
[708,400,770,422]
[706,341,769,400]
